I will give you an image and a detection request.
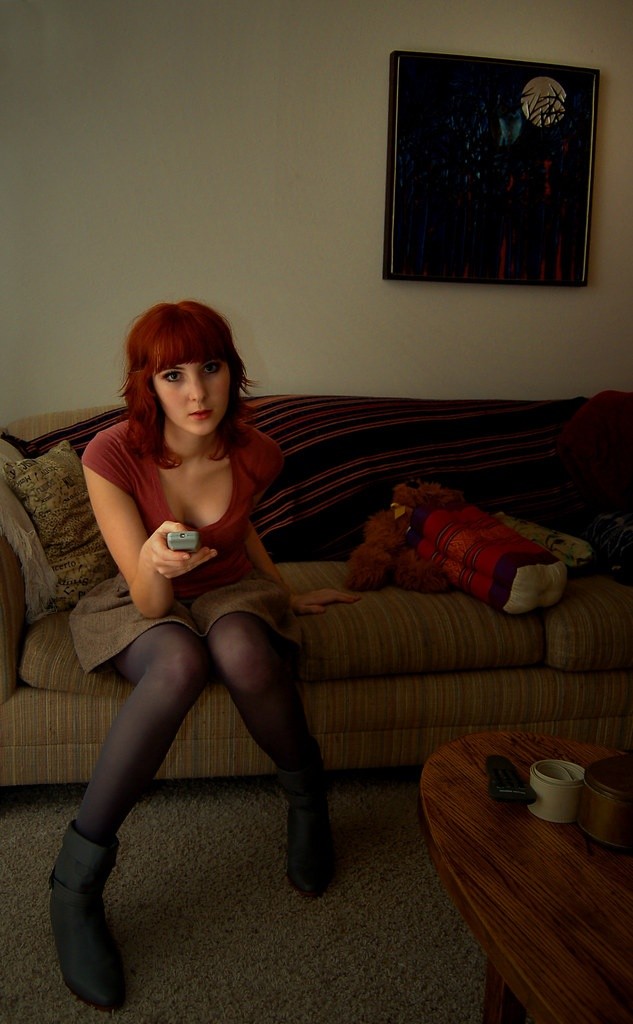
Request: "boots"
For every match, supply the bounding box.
[48,818,127,1012]
[276,736,336,898]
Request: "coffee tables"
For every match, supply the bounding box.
[420,730,633,1024]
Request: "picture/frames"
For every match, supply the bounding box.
[382,49,600,287]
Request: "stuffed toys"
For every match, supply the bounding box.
[342,479,466,595]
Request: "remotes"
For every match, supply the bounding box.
[166,531,202,553]
[486,755,538,805]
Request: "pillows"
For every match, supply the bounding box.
[401,496,568,614]
[489,510,594,579]
[4,440,119,615]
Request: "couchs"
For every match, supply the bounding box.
[0,395,633,786]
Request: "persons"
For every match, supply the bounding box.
[49,302,362,1012]
